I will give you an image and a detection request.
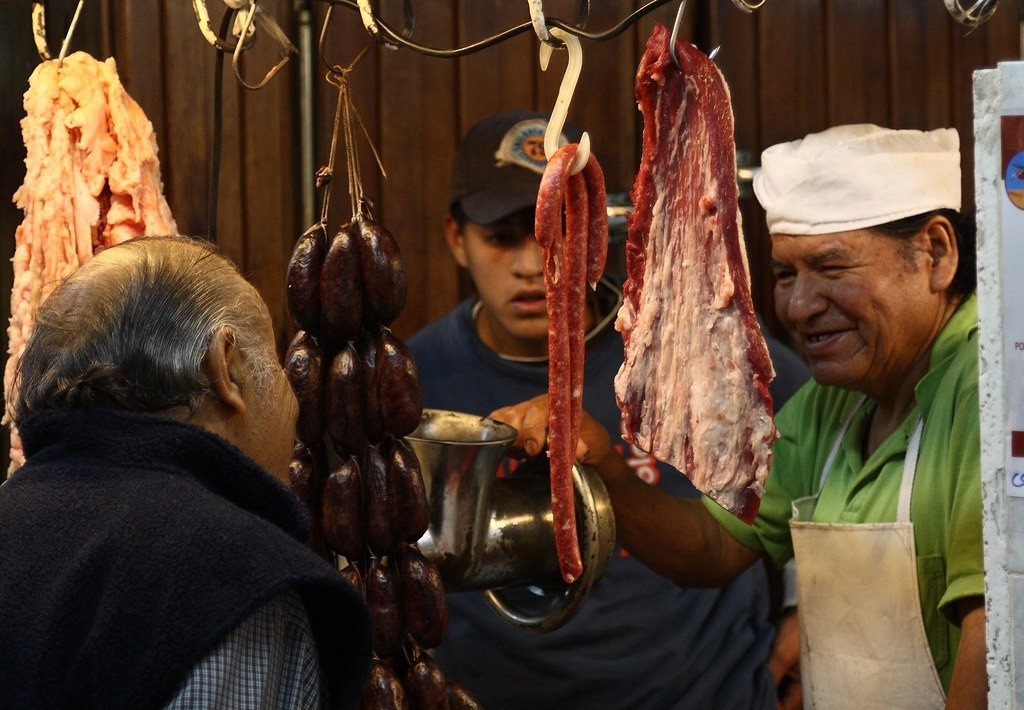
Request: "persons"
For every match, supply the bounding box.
[0,234,353,708]
[486,122,991,708]
[403,109,818,708]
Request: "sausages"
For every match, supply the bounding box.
[534,139,607,583]
[279,216,481,710]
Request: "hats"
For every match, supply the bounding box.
[752,123,962,235]
[449,113,582,227]
